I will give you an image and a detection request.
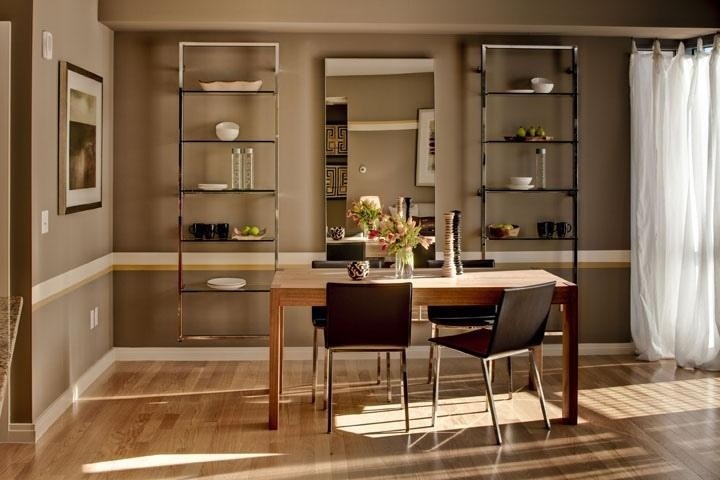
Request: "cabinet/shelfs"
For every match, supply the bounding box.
[473,42,581,287]
[177,42,280,343]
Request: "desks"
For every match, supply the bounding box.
[268,270,578,425]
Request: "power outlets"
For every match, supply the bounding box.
[42,211,49,234]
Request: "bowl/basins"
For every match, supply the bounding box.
[531,77,553,93]
[510,177,532,185]
[216,121,239,140]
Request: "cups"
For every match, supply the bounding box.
[204,224,215,240]
[189,223,203,238]
[557,222,571,237]
[547,222,557,236]
[329,226,345,240]
[347,260,369,280]
[215,224,229,239]
[537,223,545,236]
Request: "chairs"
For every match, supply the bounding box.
[312,257,391,406]
[428,281,557,445]
[427,258,496,382]
[324,282,413,432]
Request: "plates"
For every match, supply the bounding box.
[207,283,247,289]
[198,186,227,190]
[502,89,534,95]
[507,185,535,190]
[208,277,246,285]
[197,183,227,186]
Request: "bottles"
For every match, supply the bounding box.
[232,148,242,189]
[536,148,546,188]
[243,148,253,189]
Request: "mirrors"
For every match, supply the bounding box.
[323,55,434,259]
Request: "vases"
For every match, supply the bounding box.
[363,222,376,239]
[395,249,414,279]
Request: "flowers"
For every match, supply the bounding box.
[345,198,382,239]
[368,214,432,270]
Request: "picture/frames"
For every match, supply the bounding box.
[57,61,103,216]
[414,108,434,187]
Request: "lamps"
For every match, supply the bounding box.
[359,195,381,211]
